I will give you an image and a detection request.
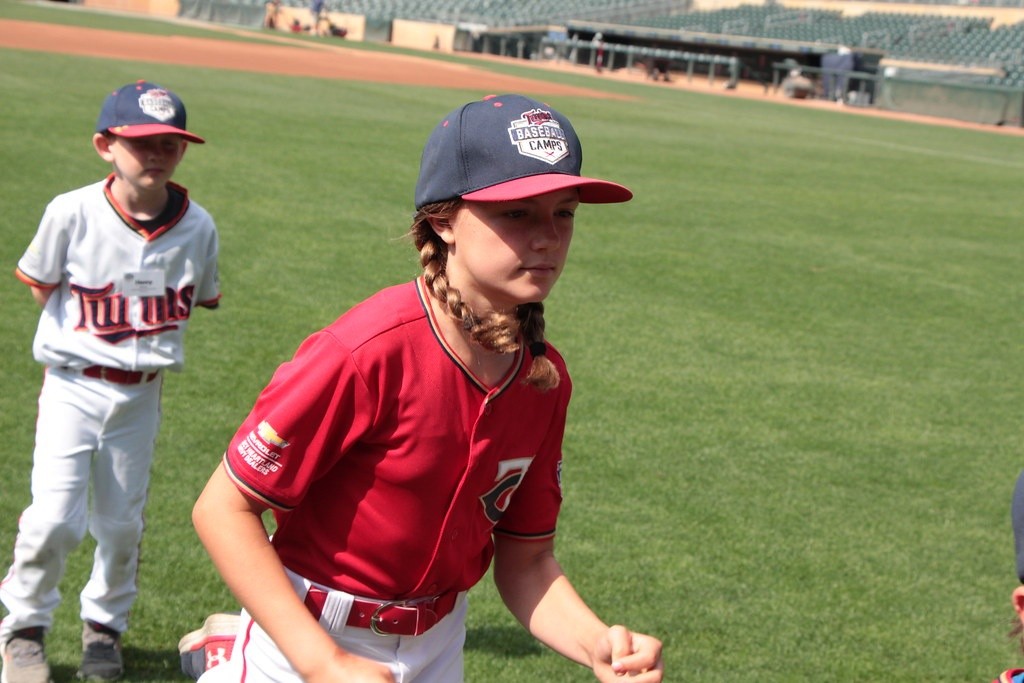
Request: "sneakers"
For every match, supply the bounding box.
[78,625,124,683]
[1,627,52,683]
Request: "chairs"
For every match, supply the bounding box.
[231,0,1024,94]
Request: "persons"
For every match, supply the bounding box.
[0,78,223,683]
[176,94,664,683]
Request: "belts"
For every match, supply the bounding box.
[80,366,162,387]
[305,584,459,639]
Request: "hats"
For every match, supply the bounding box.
[98,80,206,147]
[416,92,633,208]
